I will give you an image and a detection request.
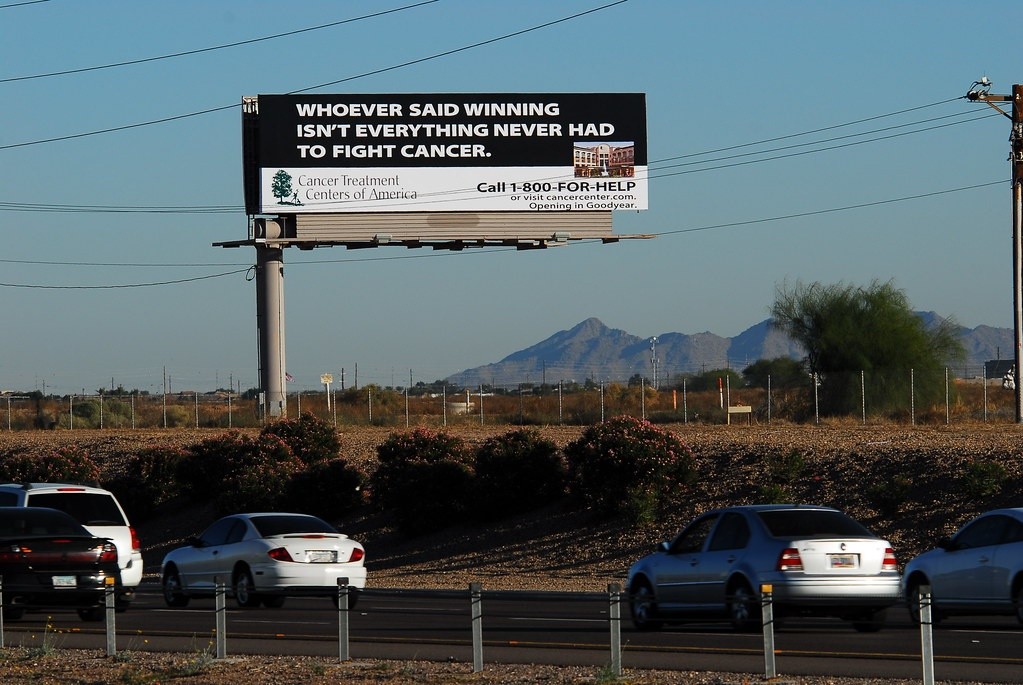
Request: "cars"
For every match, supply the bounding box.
[161,512,368,610]
[902,508,1023,628]
[626,504,902,634]
[0,506,122,622]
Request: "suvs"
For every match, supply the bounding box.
[0,480,143,614]
